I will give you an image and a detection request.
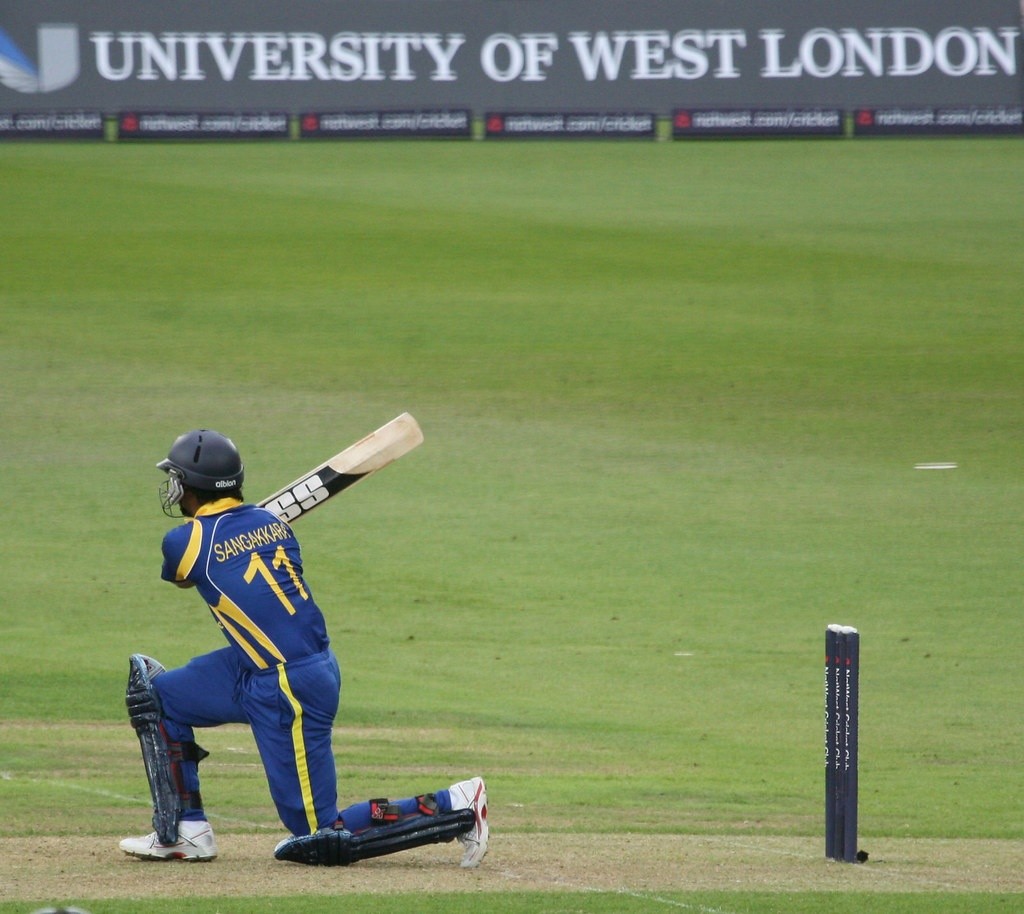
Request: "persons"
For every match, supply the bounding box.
[120,430,489,869]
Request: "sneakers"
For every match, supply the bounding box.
[119,820,217,862]
[449,776,488,869]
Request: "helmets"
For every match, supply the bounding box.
[155,430,244,491]
